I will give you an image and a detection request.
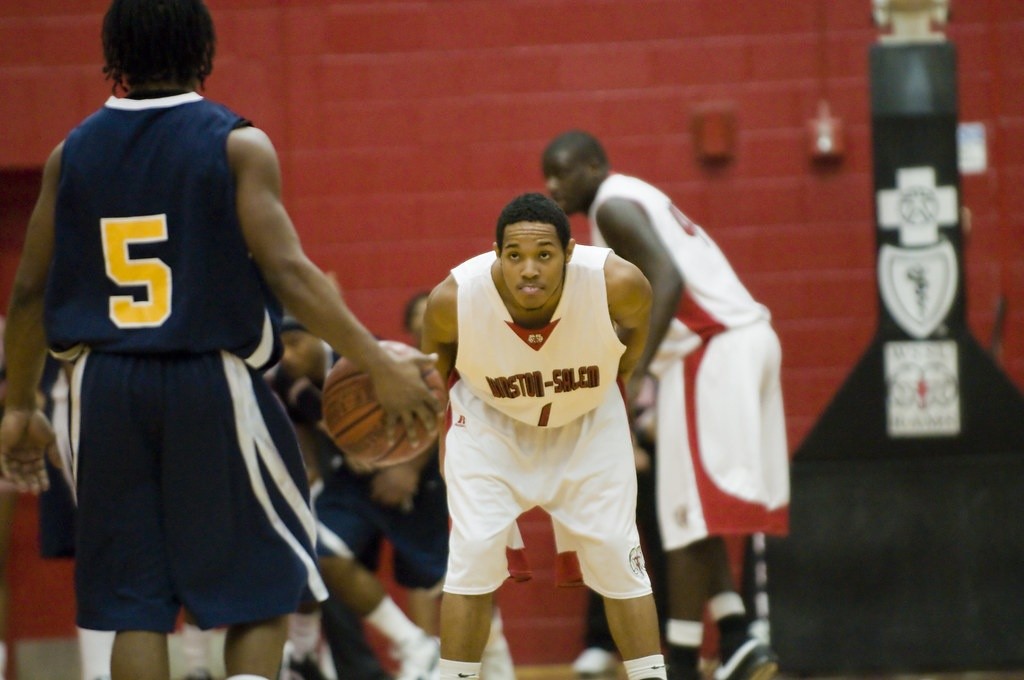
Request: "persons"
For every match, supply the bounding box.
[0,0,447,680]
[36,130,788,680]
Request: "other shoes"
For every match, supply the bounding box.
[389,631,442,680]
[280,638,339,680]
[574,645,612,674]
[721,638,777,680]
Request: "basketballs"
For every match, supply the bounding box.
[321,342,450,467]
